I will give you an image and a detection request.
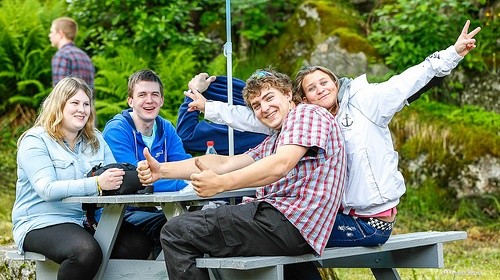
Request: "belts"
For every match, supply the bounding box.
[367,217,396,231]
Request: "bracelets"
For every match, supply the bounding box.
[188,89,200,94]
[96,177,103,196]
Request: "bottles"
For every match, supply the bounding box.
[205,141,217,154]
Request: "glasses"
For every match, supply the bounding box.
[247,71,272,83]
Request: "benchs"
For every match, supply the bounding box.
[4,251,47,260]
[196,229,467,280]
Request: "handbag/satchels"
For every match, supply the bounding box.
[81,162,146,233]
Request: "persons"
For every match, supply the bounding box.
[178,72,269,205]
[11,75,153,280]
[135,68,347,280]
[94,70,195,260]
[49,17,94,99]
[184,20,480,280]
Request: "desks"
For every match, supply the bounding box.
[36,188,256,280]
